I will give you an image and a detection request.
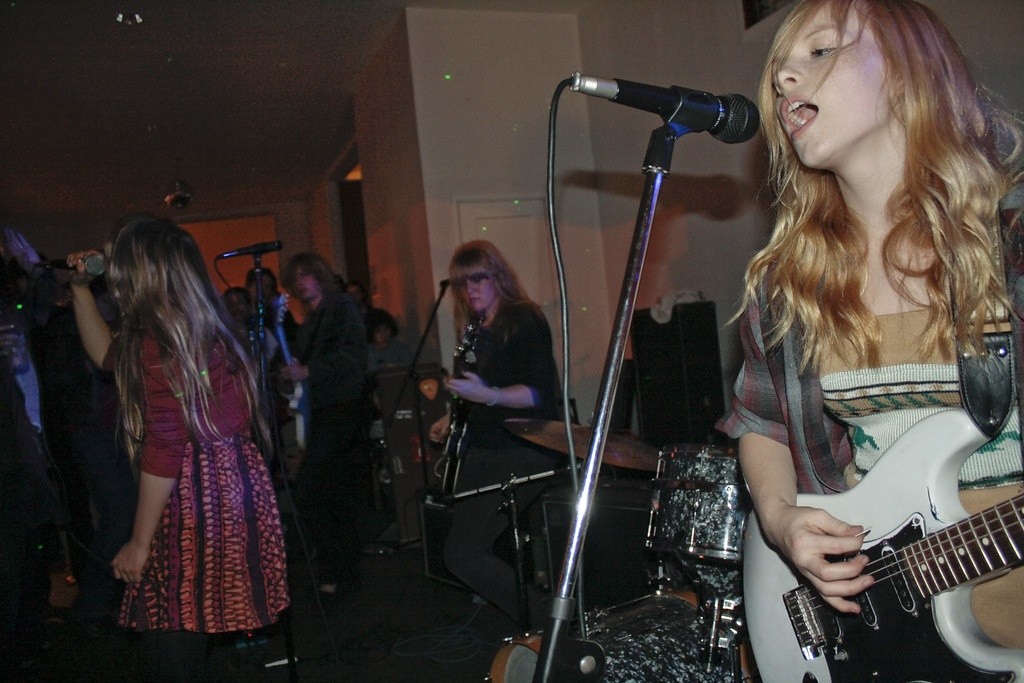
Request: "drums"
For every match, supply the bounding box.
[483,589,762,683]
[638,443,745,563]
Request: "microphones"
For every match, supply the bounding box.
[219,240,283,258]
[568,71,761,145]
[50,254,107,276]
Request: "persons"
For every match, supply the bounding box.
[2,225,411,672]
[282,253,364,580]
[428,241,566,636]
[68,215,273,683]
[718,2,1022,682]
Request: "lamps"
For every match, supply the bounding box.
[162,175,193,209]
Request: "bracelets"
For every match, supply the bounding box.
[486,387,500,406]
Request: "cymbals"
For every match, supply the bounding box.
[503,416,664,470]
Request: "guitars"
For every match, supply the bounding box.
[274,292,311,454]
[741,406,1024,683]
[439,313,485,495]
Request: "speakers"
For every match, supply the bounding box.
[630,300,726,450]
[539,479,660,618]
[417,488,517,591]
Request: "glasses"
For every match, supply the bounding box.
[452,273,496,286]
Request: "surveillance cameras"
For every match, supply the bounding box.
[165,192,191,208]
